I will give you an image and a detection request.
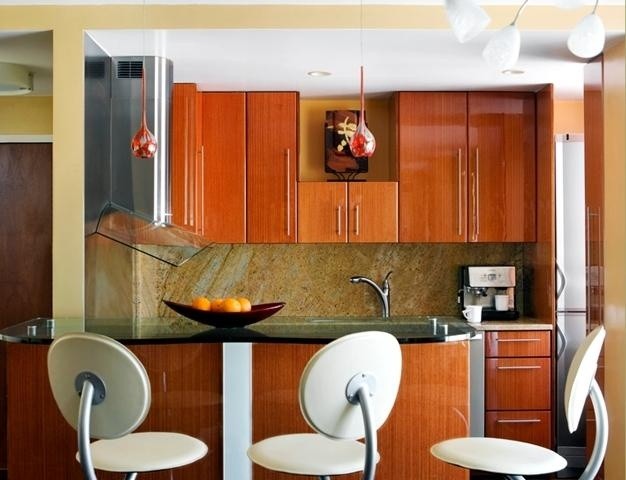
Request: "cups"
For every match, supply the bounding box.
[461,304,483,324]
[495,295,509,311]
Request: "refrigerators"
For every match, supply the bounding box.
[556,129,588,477]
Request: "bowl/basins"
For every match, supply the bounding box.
[160,298,287,328]
[191,327,267,337]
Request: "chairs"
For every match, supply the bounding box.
[47,334,211,480]
[250,331,405,480]
[432,326,610,479]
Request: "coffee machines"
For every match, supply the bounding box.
[457,263,520,321]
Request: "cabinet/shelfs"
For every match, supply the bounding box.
[202,92,296,243]
[397,92,539,244]
[485,329,554,449]
[299,180,398,245]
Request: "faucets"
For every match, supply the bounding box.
[348,269,395,319]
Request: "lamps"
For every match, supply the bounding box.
[442,0,606,76]
[348,0,376,160]
[129,2,159,161]
